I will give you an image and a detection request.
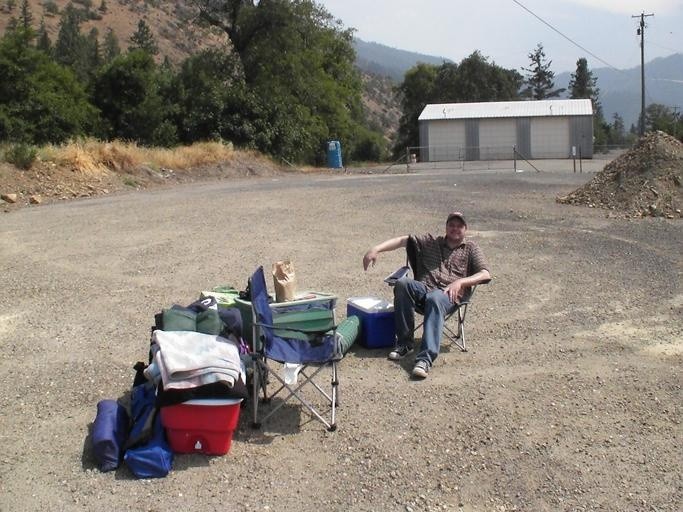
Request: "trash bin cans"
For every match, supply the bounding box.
[411,154,417,164]
[326,141,343,168]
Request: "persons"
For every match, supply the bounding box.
[362,211,492,378]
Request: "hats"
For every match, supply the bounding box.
[447,211,467,227]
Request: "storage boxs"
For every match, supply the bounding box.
[161,398,243,454]
[234,288,396,349]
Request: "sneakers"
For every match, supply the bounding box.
[389,344,415,361]
[411,359,430,378]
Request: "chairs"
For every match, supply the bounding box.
[386,245,479,352]
[248,265,341,432]
